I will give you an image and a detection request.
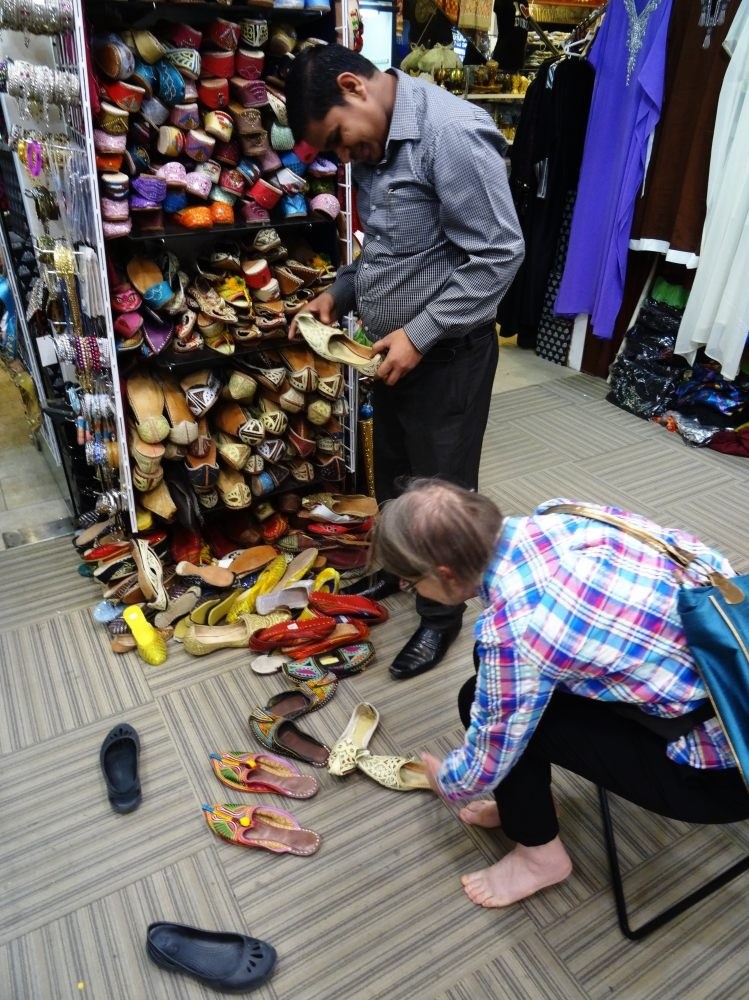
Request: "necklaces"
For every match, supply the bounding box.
[696,0,729,50]
[621,0,661,87]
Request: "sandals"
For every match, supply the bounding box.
[145,921,278,993]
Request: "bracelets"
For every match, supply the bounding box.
[0,0,133,518]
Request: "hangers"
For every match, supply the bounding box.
[528,21,595,91]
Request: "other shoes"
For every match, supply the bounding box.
[69,16,463,855]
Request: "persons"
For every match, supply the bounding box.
[286,41,526,682]
[371,479,747,909]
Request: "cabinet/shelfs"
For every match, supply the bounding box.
[0,0,398,606]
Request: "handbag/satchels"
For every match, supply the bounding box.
[540,504,749,792]
